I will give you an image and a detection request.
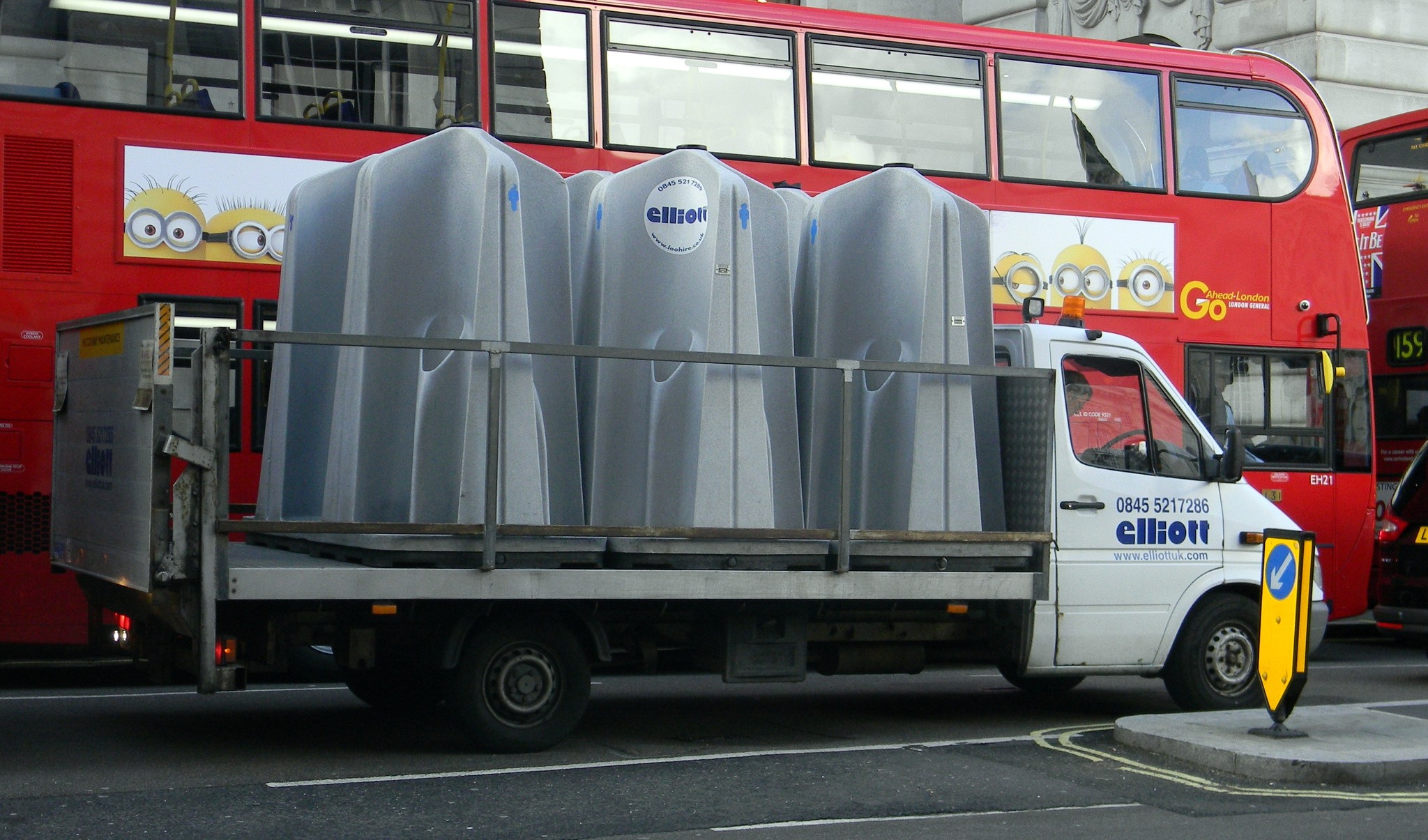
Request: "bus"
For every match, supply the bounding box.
[0,2,1427,631]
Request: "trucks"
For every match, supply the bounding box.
[45,298,1331,756]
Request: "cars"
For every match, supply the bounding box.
[1371,435,1428,637]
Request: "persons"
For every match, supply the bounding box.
[1063,368,1093,418]
[1214,363,1268,464]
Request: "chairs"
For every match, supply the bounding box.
[435,102,474,126]
[164,78,216,111]
[302,90,361,124]
[1176,143,1281,198]
